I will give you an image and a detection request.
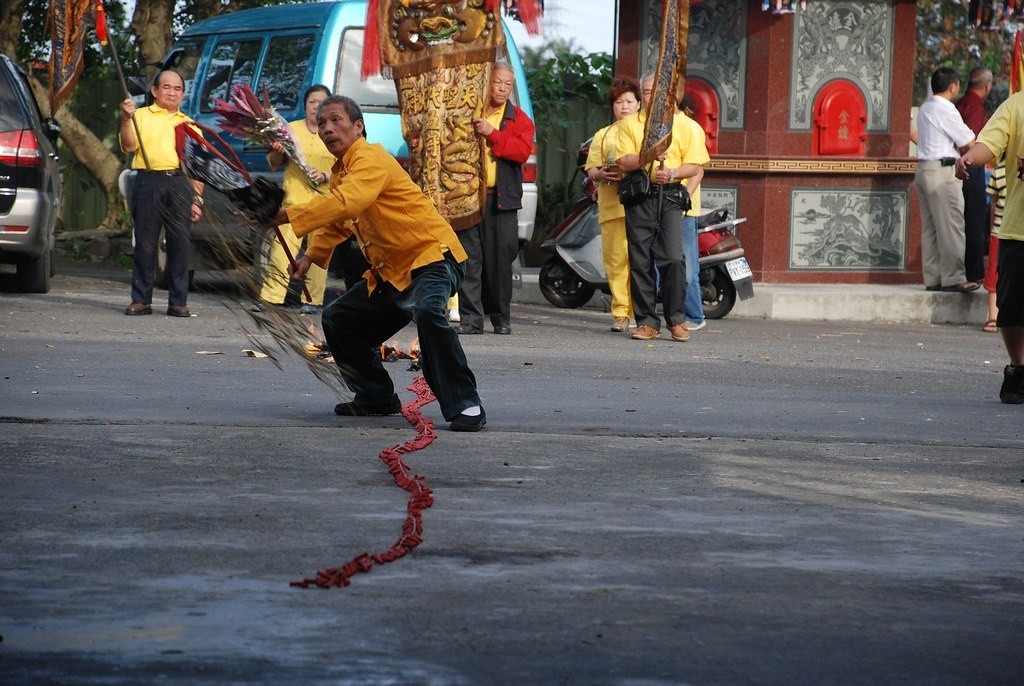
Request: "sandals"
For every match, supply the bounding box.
[926,281,980,292]
[982,319,998,332]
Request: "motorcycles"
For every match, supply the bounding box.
[538,135,755,318]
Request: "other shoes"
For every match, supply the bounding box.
[301,304,318,313]
[450,405,487,432]
[1000,364,1024,404]
[334,393,401,415]
[250,300,280,311]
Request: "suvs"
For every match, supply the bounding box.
[0,54,65,291]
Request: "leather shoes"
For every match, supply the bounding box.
[493,325,511,334]
[126,302,153,315]
[454,324,483,335]
[166,304,190,317]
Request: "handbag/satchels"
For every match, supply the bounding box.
[617,168,650,206]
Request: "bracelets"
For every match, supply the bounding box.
[669,169,674,182]
[593,172,600,183]
[321,172,328,184]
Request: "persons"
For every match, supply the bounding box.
[235,95,487,431]
[613,70,711,341]
[955,90,1024,404]
[119,70,207,317]
[585,75,640,332]
[453,62,535,334]
[250,84,337,314]
[909,69,1006,333]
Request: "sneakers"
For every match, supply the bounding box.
[631,326,661,339]
[666,321,690,342]
[686,320,706,330]
[611,316,630,331]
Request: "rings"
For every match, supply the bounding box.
[608,181,612,185]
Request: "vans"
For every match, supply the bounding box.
[118,0,538,294]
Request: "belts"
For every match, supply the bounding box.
[139,168,184,176]
